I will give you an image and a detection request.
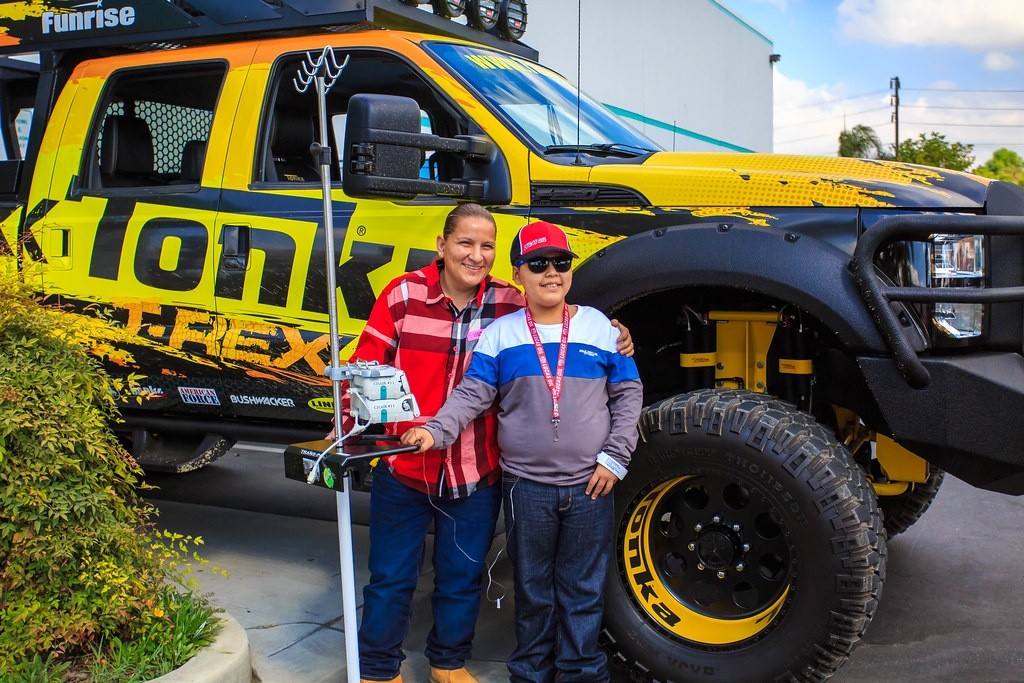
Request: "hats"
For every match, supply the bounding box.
[510,222,579,266]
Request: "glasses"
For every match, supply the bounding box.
[517,255,573,272]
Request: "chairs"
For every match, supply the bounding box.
[168,140,207,185]
[100,115,170,188]
[439,118,468,181]
[265,103,322,182]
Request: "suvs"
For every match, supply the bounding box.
[1,0,1020,683]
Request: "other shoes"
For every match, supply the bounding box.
[361,674,402,683]
[430,666,478,683]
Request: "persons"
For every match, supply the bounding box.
[327,202,531,683]
[399,220,644,682]
